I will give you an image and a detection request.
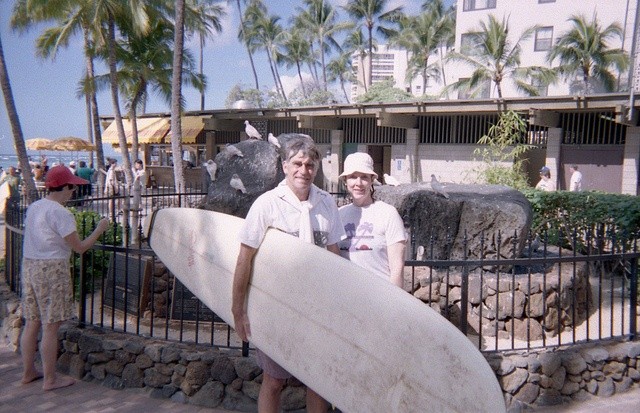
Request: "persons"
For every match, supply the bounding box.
[535,166,556,192]
[34,165,42,179]
[105,157,111,172]
[0,166,19,207]
[231,137,348,412]
[132,159,146,228]
[75,161,95,195]
[570,166,582,191]
[337,153,405,288]
[105,159,118,224]
[43,153,49,176]
[69,161,76,175]
[21,164,110,390]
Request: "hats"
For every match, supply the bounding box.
[338,152,378,183]
[70,161,77,165]
[46,165,88,188]
[539,166,550,175]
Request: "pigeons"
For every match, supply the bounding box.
[429,174,450,200]
[383,173,400,187]
[224,143,247,158]
[206,159,218,182]
[243,119,263,140]
[267,132,280,147]
[229,172,248,196]
